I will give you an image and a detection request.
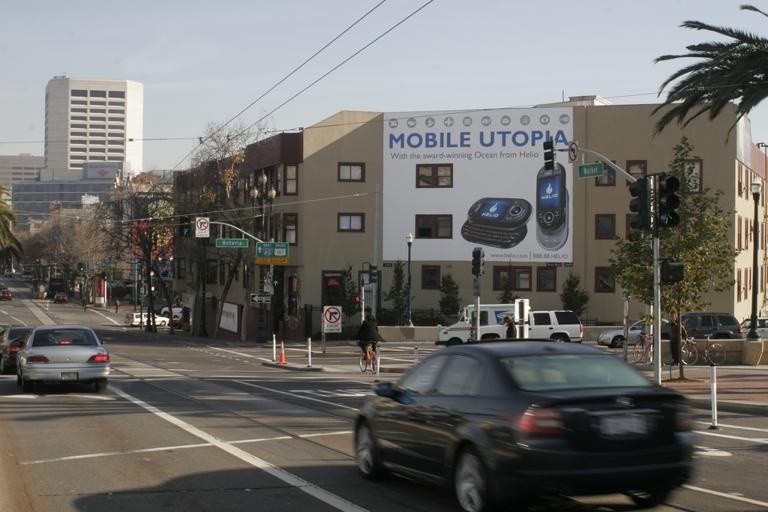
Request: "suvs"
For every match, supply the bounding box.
[675,311,744,339]
[481,309,583,344]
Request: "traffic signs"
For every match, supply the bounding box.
[255,242,289,257]
[250,293,271,303]
[576,162,603,179]
[216,238,249,248]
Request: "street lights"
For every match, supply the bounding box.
[747,176,762,338]
[250,168,277,343]
[404,233,414,326]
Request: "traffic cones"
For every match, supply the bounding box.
[277,340,288,364]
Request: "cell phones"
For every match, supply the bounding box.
[461,198,532,248]
[536,162,569,251]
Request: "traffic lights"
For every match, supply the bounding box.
[658,175,680,232]
[369,263,378,284]
[543,136,554,175]
[480,250,486,276]
[470,249,480,276]
[354,295,361,304]
[629,178,649,232]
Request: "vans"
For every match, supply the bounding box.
[434,303,531,347]
[124,312,170,327]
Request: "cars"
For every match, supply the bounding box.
[0,327,37,374]
[14,324,112,392]
[160,300,183,318]
[739,318,767,340]
[353,340,695,511]
[0,324,25,333]
[597,317,687,348]
[0,265,70,304]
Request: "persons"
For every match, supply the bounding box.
[504,316,517,340]
[669,311,688,366]
[114,298,120,313]
[82,294,86,305]
[356,315,387,360]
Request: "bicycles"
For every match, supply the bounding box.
[355,335,380,374]
[632,333,654,366]
[681,328,727,366]
